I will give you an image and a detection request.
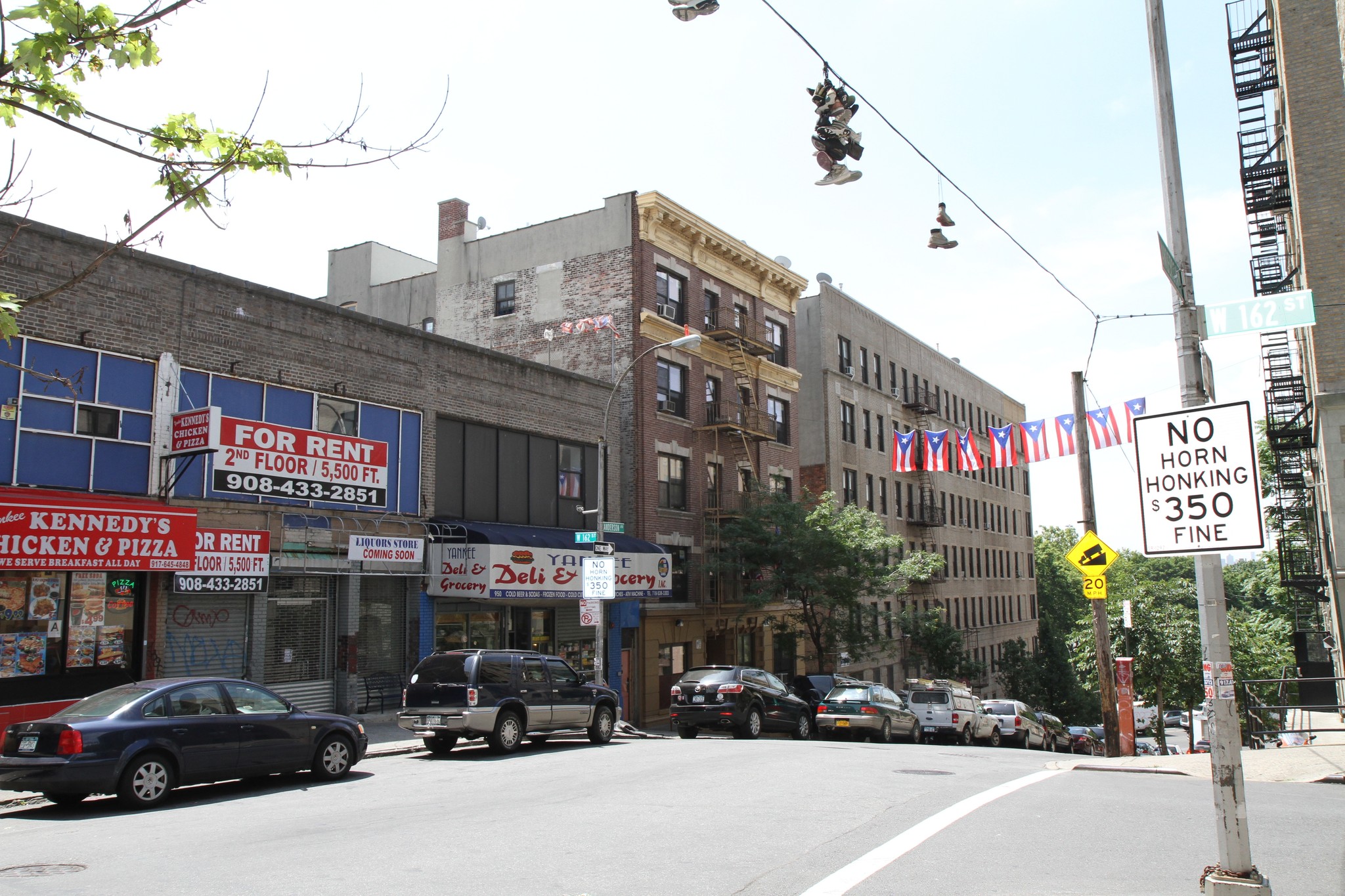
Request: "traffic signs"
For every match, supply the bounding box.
[593,540,615,557]
[579,599,601,626]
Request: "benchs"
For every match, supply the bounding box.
[363,672,405,714]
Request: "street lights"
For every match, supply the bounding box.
[593,333,705,689]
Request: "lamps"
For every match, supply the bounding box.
[675,619,684,627]
[1322,636,1336,648]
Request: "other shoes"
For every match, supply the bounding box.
[806,79,863,186]
[668,0,721,21]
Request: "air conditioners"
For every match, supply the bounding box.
[961,572,964,577]
[959,518,967,525]
[962,421,965,427]
[657,304,675,320]
[925,404,928,410]
[1016,571,1022,576]
[657,400,675,412]
[845,366,855,376]
[705,323,715,331]
[984,523,990,528]
[891,388,900,396]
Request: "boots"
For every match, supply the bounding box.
[936,202,955,227]
[927,229,958,249]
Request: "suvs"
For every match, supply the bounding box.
[1179,706,1204,730]
[1033,711,1074,754]
[980,698,1047,751]
[396,648,623,756]
[906,683,1002,748]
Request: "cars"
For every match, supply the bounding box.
[791,675,922,745]
[1162,709,1184,729]
[1134,740,1211,757]
[671,665,813,740]
[1066,726,1105,757]
[0,677,370,814]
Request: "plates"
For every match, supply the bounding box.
[49,591,59,598]
[31,582,51,599]
[80,628,95,666]
[29,596,57,619]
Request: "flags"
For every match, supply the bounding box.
[890,397,1146,473]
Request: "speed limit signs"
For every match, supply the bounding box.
[1082,575,1108,599]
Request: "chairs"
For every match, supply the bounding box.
[180,693,196,709]
[201,698,222,714]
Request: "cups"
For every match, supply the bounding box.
[116,634,123,639]
[70,603,85,625]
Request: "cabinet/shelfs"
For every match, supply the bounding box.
[531,608,555,656]
[558,638,596,682]
[435,612,499,652]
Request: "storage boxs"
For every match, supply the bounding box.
[559,643,594,666]
